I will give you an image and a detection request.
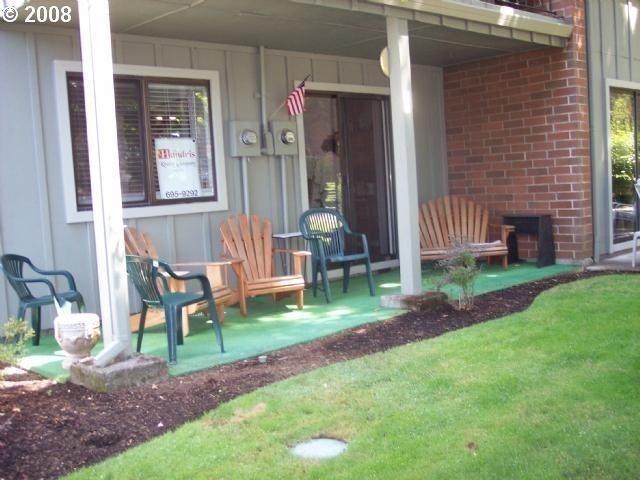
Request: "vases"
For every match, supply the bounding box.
[54,313,101,370]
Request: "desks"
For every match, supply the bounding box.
[502,214,555,268]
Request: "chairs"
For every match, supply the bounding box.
[124,254,227,367]
[0,254,86,346]
[219,213,313,318]
[299,207,376,305]
[123,222,246,337]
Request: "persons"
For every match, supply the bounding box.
[311,136,340,211]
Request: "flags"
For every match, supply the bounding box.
[286,81,306,116]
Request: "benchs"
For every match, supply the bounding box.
[419,195,516,270]
